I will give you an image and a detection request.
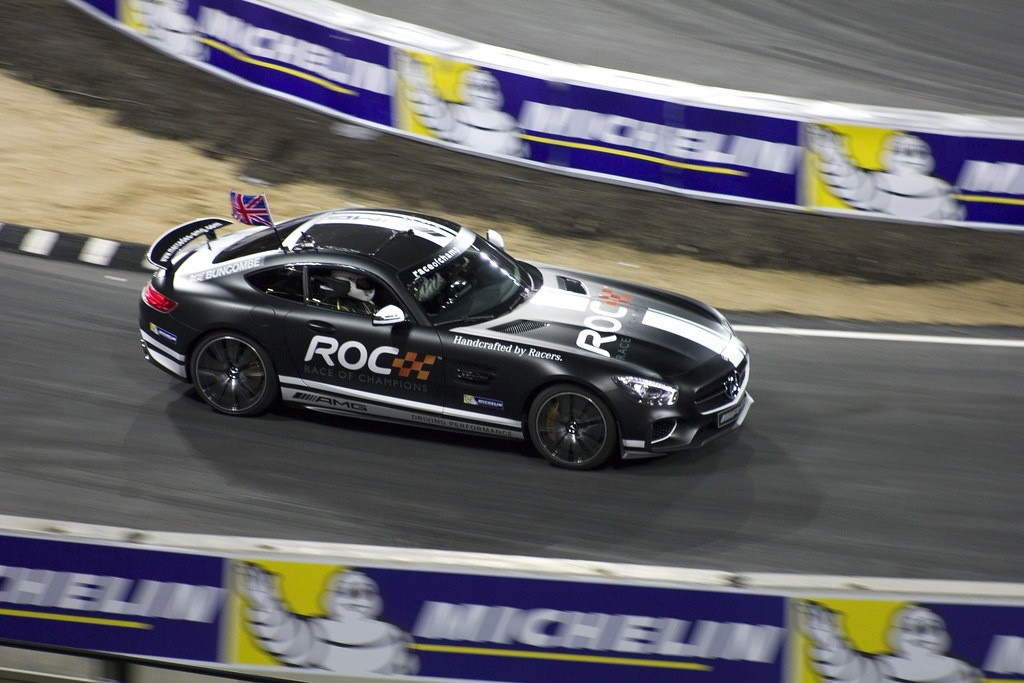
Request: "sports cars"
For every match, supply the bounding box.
[139,207,756,471]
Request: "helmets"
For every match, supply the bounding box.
[331,268,375,301]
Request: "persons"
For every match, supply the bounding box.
[322,269,378,315]
[414,256,469,314]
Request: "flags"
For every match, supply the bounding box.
[230,192,275,229]
